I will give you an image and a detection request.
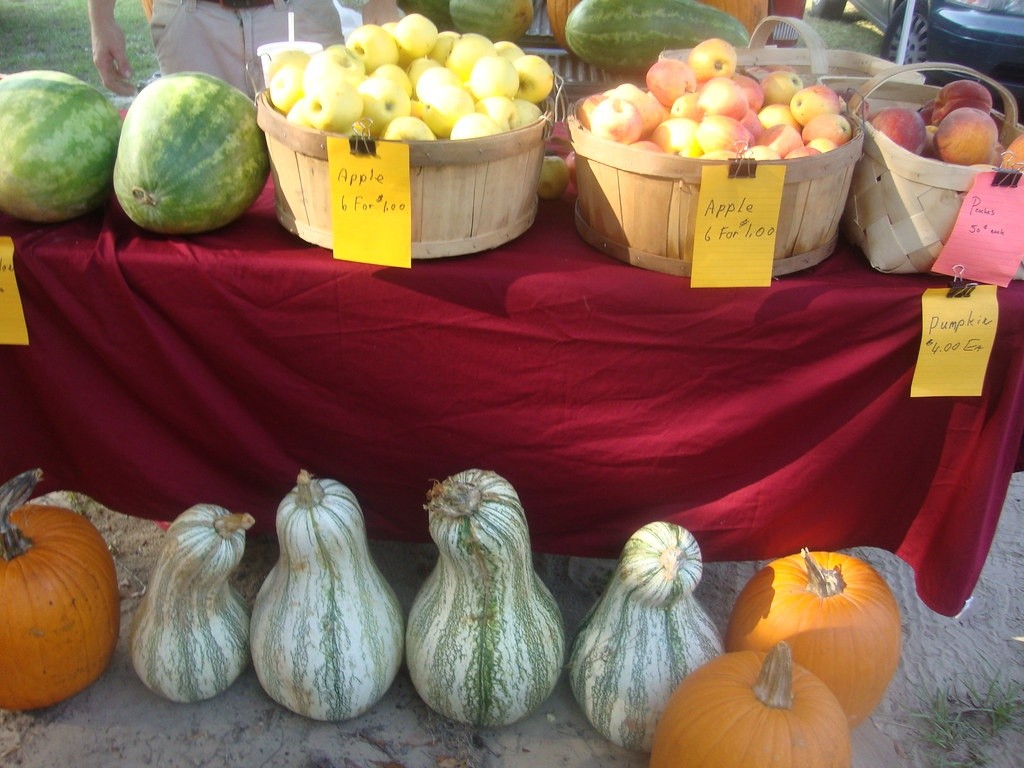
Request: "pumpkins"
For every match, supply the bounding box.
[1,469,901,768]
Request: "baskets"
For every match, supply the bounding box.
[657,16,926,90]
[818,62,1023,275]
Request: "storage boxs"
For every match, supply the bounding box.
[257,92,555,257]
[845,62,1024,273]
[567,98,865,276]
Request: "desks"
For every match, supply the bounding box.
[0,109,1024,618]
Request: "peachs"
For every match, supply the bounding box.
[838,78,1024,170]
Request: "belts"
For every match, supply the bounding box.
[199,0,273,8]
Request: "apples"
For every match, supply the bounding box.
[271,13,547,142]
[575,41,851,164]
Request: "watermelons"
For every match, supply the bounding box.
[395,0,534,41]
[0,70,269,234]
[564,0,748,73]
